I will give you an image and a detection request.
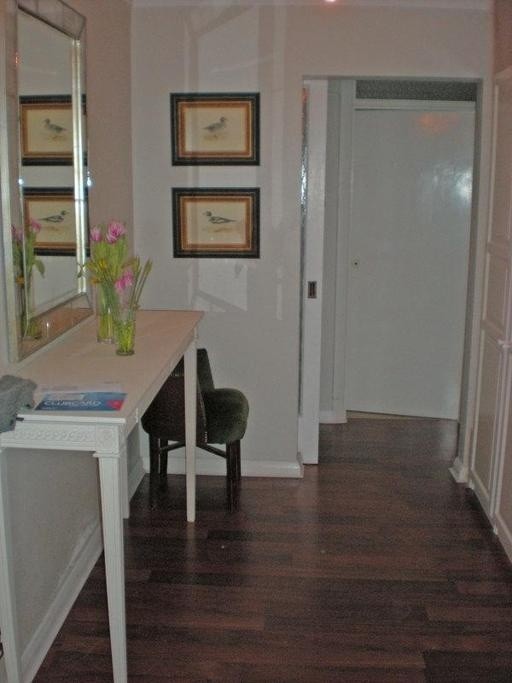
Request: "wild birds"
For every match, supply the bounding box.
[38,209,70,231]
[202,210,234,230]
[40,116,66,140]
[202,114,229,138]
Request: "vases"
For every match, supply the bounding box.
[102,282,139,357]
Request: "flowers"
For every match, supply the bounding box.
[9,217,47,338]
[76,213,154,353]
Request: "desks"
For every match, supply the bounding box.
[0,308,207,683]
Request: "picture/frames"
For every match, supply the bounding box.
[168,91,262,258]
[19,95,92,255]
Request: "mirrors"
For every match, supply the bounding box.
[1,1,96,363]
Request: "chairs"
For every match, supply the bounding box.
[141,348,250,514]
